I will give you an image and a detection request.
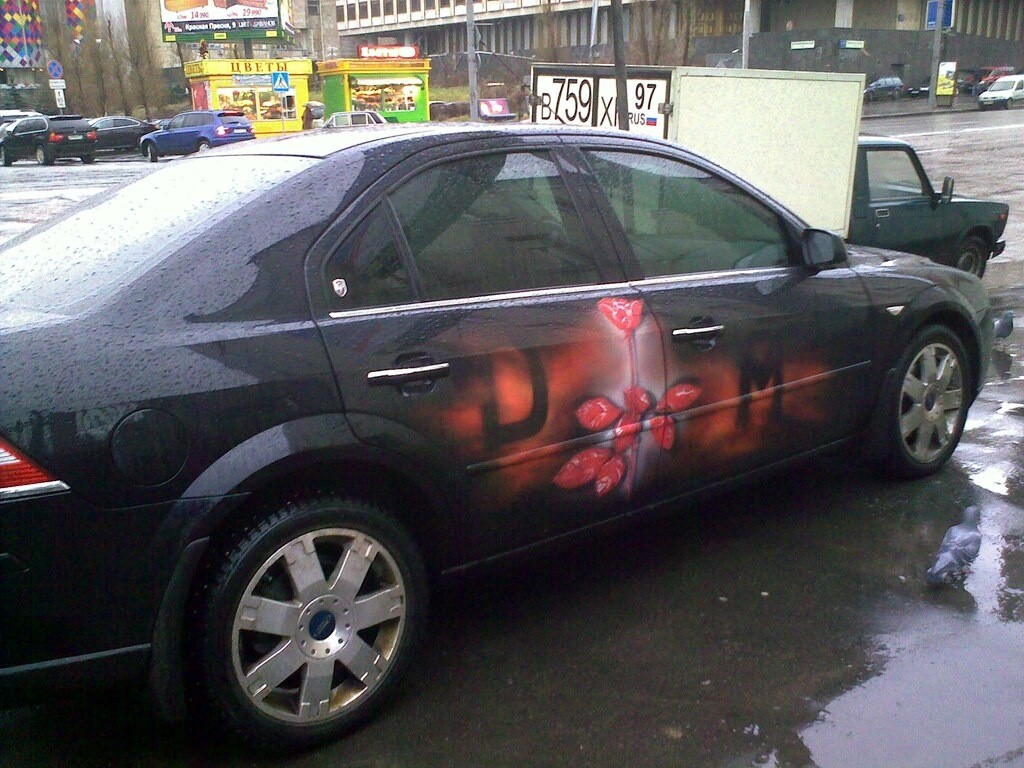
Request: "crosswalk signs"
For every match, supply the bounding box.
[271,72,290,92]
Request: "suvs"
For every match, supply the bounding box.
[139,109,259,161]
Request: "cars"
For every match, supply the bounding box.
[155,118,172,126]
[85,118,159,149]
[0,123,997,767]
[978,75,1024,110]
[907,74,965,99]
[0,110,42,142]
[0,115,97,168]
[863,74,904,103]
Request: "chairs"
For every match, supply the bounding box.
[464,182,583,293]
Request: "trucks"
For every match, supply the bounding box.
[528,62,1009,281]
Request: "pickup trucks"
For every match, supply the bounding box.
[964,66,1008,94]
